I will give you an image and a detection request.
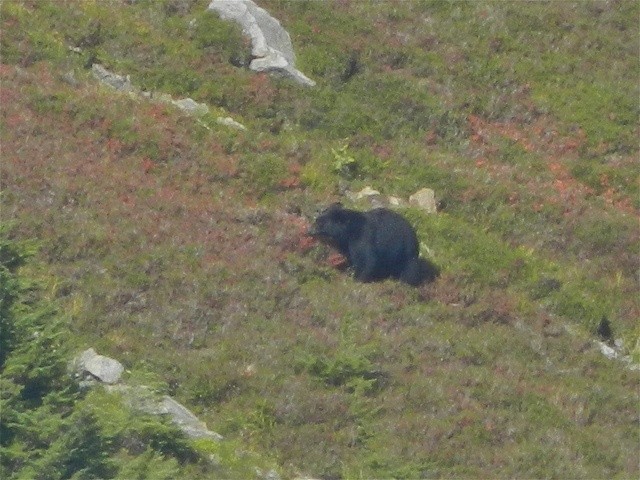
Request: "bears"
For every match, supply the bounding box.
[307,202,441,284]
